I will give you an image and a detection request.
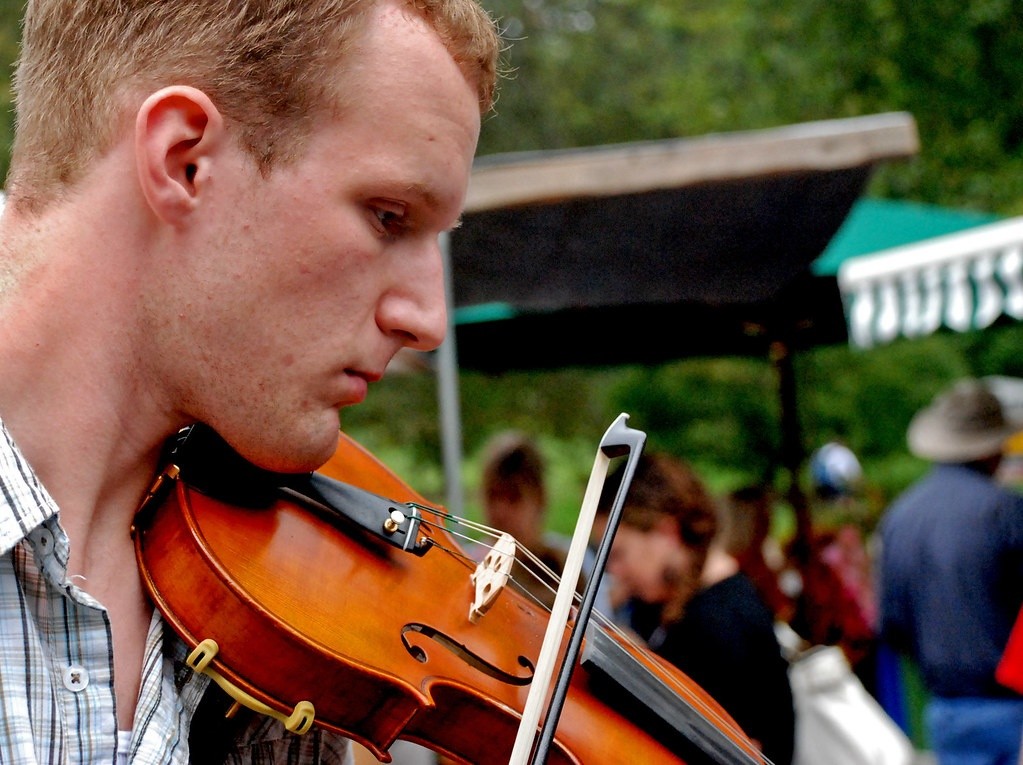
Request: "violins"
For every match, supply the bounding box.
[129,401,782,765]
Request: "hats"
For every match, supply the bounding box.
[906,375,1023,464]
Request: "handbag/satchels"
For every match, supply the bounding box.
[773,620,917,765]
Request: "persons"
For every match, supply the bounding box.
[584,454,880,765]
[874,377,1023,765]
[0,1,498,763]
[460,439,585,603]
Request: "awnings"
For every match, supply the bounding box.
[840,214,1023,346]
